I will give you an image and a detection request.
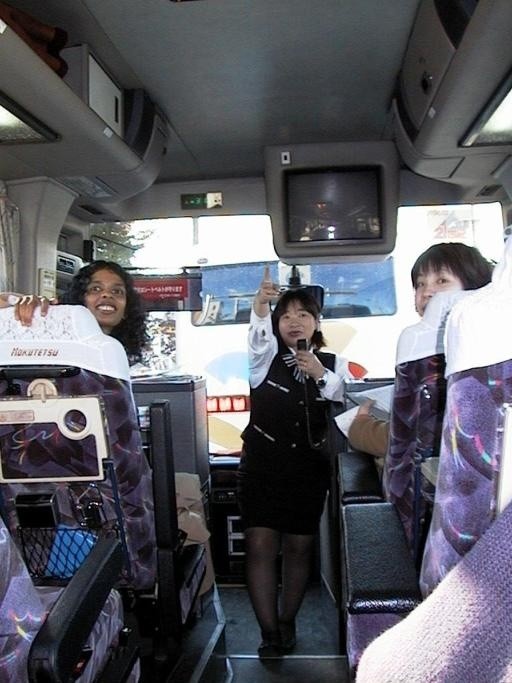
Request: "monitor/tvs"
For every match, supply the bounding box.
[278,163,386,246]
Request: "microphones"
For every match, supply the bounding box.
[297,339,313,386]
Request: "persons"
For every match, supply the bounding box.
[12,260,147,359]
[238,263,347,659]
[344,242,494,496]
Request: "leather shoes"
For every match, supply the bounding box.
[258,622,296,654]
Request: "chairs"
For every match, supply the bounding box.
[342,234,512,681]
[338,291,474,582]
[1,304,207,633]
[1,518,142,682]
